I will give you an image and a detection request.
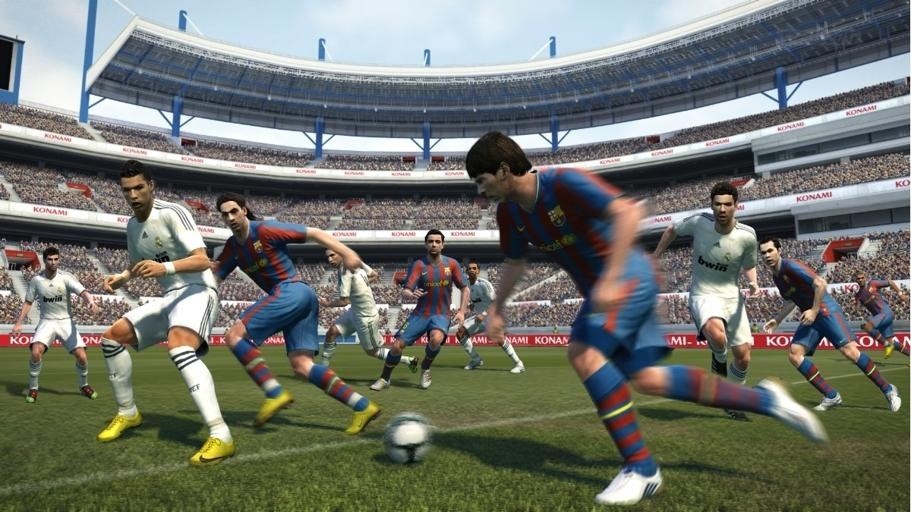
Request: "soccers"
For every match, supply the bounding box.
[382,413,437,465]
